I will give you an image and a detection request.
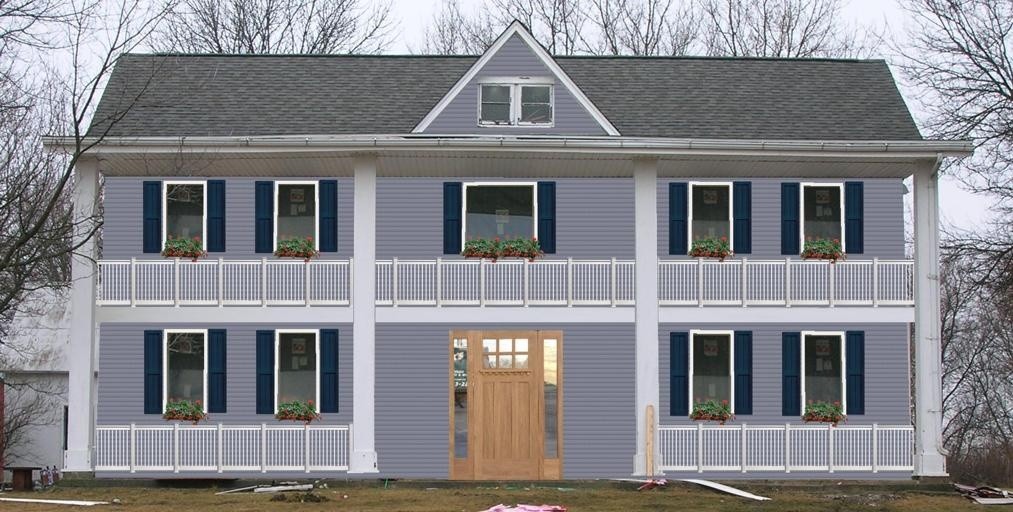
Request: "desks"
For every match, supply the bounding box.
[3,466,44,489]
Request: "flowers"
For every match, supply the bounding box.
[277,400,320,423]
[463,235,545,263]
[162,396,208,424]
[689,236,736,260]
[273,234,322,259]
[802,400,847,425]
[800,236,850,263]
[686,400,733,420]
[161,236,204,262]
